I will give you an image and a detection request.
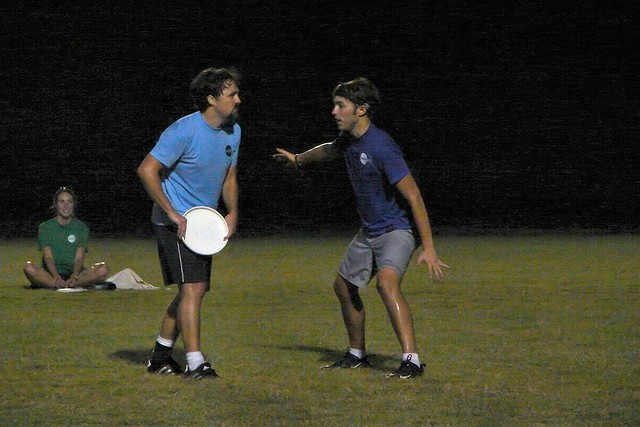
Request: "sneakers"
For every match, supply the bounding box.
[181,362,222,381]
[319,346,371,371]
[94,282,116,291]
[146,356,185,376]
[385,354,426,381]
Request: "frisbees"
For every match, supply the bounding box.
[181,206,229,256]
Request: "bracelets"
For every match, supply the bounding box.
[295,153,301,170]
[71,274,78,281]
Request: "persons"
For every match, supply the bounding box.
[23,187,109,290]
[271,77,452,381]
[137,68,243,384]
